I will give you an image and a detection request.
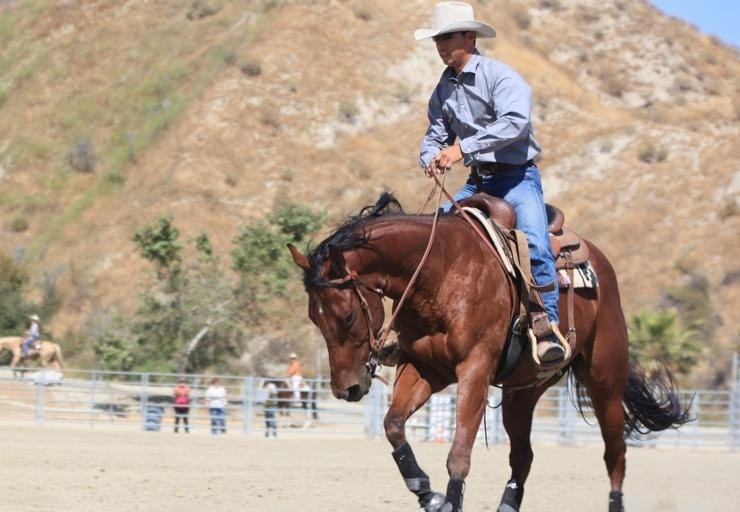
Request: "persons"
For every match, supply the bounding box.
[413,1,567,380]
[205,378,229,434]
[21,314,40,360]
[258,383,280,437]
[146,398,164,430]
[172,377,194,434]
[278,351,303,407]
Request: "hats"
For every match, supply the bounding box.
[414,1,496,39]
[30,315,40,322]
[291,352,296,357]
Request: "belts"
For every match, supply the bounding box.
[470,160,535,175]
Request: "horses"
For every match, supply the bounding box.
[286,188,700,512]
[259,374,318,428]
[0,337,66,378]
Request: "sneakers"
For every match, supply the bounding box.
[535,334,565,381]
